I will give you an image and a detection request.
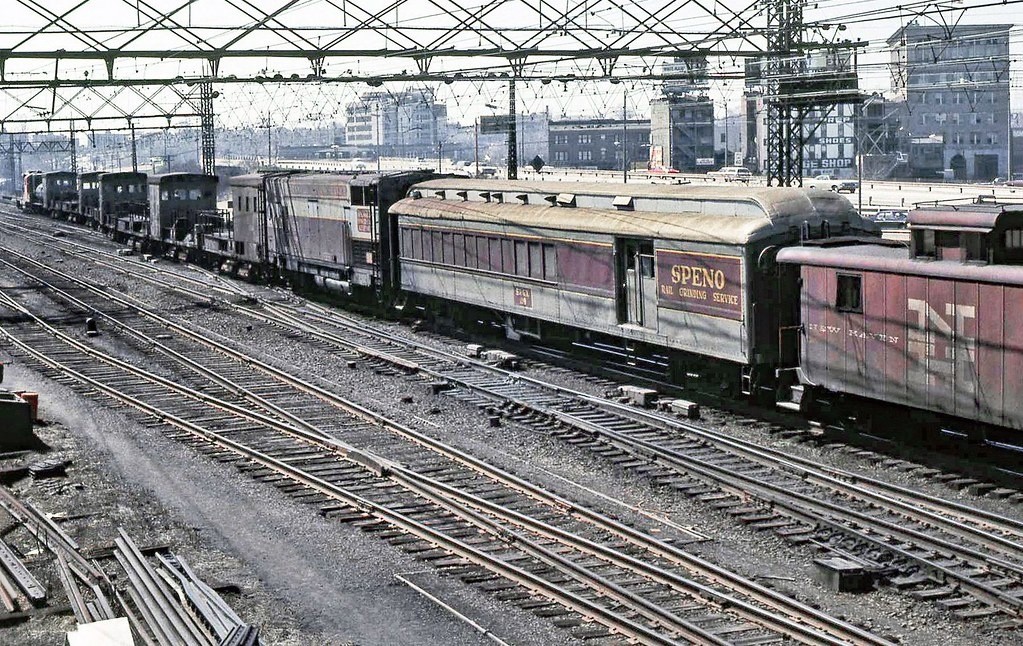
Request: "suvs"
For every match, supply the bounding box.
[706,167,752,182]
[1007,177,1023,187]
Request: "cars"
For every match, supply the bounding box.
[645,168,679,179]
[803,175,858,193]
[524,165,554,174]
[980,177,1007,185]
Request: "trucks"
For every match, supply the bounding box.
[463,162,497,176]
[456,160,471,171]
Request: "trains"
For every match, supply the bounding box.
[16,173,1023,434]
